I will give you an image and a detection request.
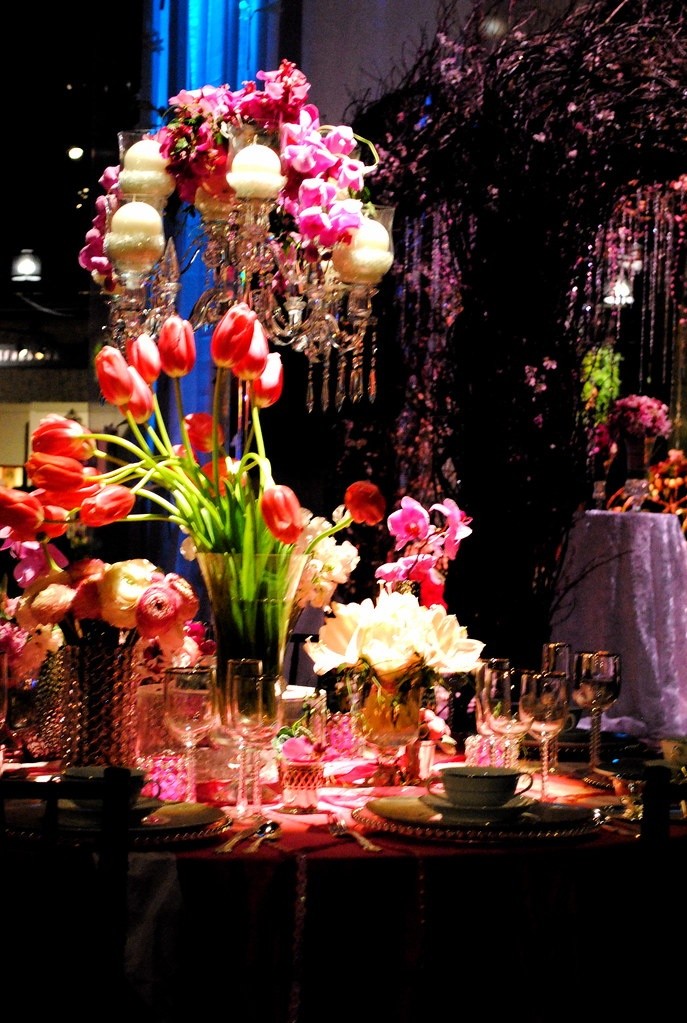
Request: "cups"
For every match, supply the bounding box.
[403,741,435,780]
[49,766,161,810]
[426,766,534,806]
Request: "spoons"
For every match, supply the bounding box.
[244,821,280,853]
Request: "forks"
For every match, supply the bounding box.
[327,814,383,852]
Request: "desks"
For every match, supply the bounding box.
[122,761,687,1023]
[550,509,687,745]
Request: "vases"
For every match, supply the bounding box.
[196,551,310,728]
[353,673,422,788]
[625,436,655,513]
[64,644,141,769]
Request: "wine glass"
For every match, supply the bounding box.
[474,640,622,806]
[162,657,277,826]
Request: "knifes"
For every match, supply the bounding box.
[213,823,263,853]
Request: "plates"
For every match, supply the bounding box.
[4,798,226,833]
[41,796,166,828]
[419,793,539,822]
[366,796,595,831]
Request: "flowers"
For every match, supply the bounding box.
[14,555,200,648]
[0,303,388,554]
[303,590,486,680]
[607,395,672,444]
[78,59,382,294]
